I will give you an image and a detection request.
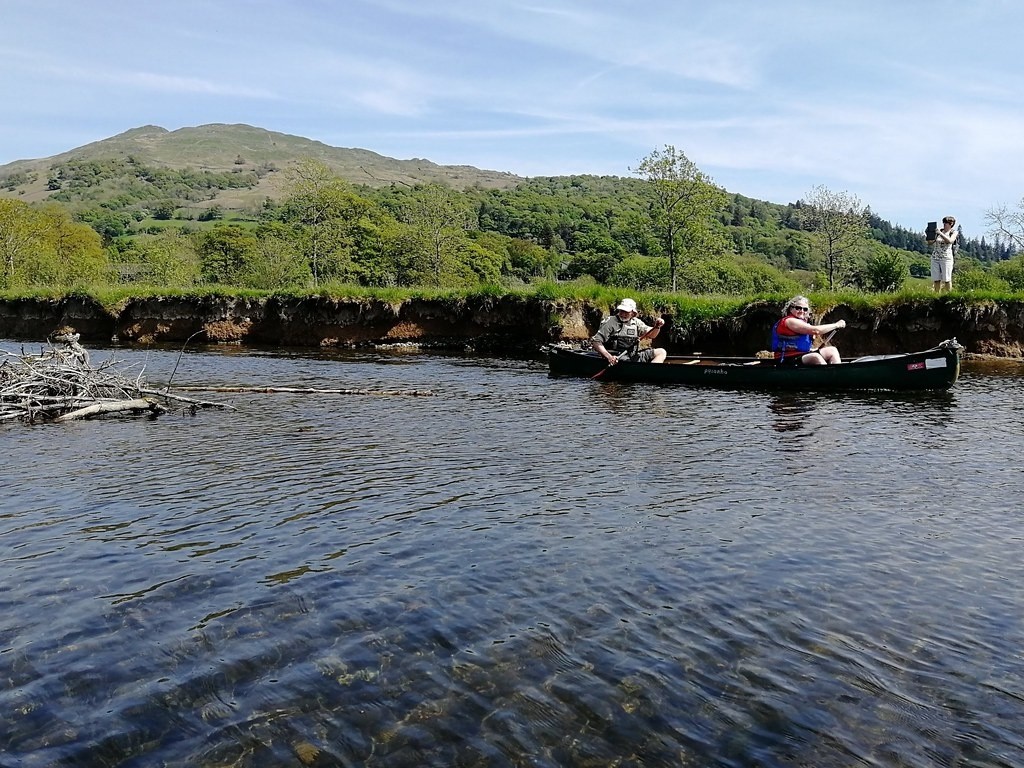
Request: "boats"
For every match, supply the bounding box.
[548,336,965,394]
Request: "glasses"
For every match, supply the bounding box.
[790,306,808,311]
[942,219,951,224]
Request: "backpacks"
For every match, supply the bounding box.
[949,228,960,256]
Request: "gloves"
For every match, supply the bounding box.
[935,228,942,235]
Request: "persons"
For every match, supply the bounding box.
[932,215,958,292]
[590,297,667,365]
[776,295,847,365]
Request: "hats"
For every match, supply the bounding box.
[616,298,636,312]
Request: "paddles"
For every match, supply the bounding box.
[814,325,839,353]
[592,321,660,380]
